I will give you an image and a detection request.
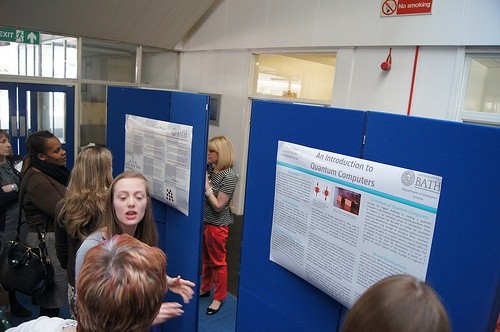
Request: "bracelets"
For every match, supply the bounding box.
[205,188,213,197]
[12,183,19,191]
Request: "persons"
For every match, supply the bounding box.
[342,274,452,332]
[0,129,195,332]
[199,136,238,315]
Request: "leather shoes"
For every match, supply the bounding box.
[206,300,223,315]
[199,291,210,298]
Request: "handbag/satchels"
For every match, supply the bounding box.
[1,221,55,298]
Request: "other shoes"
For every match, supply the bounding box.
[30,296,39,305]
[10,304,33,318]
